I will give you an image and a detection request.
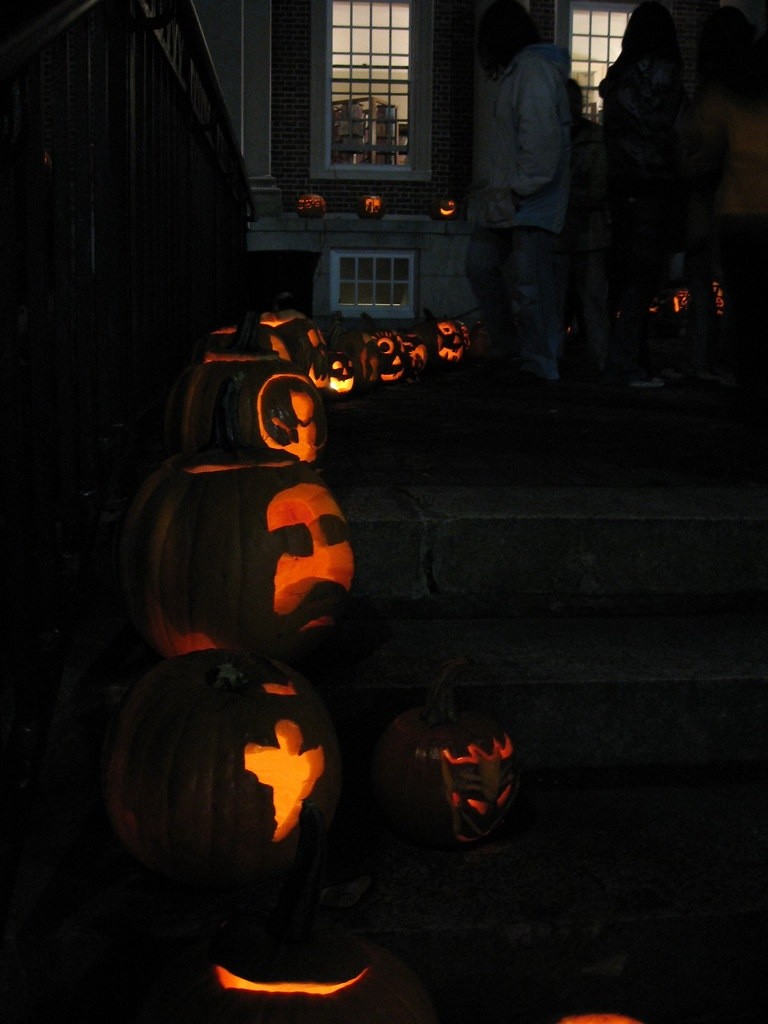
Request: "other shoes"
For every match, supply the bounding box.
[504,370,546,386]
[621,370,664,387]
[655,365,683,379]
[720,374,742,388]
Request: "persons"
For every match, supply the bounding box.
[567,0,768,387]
[466,0,574,391]
